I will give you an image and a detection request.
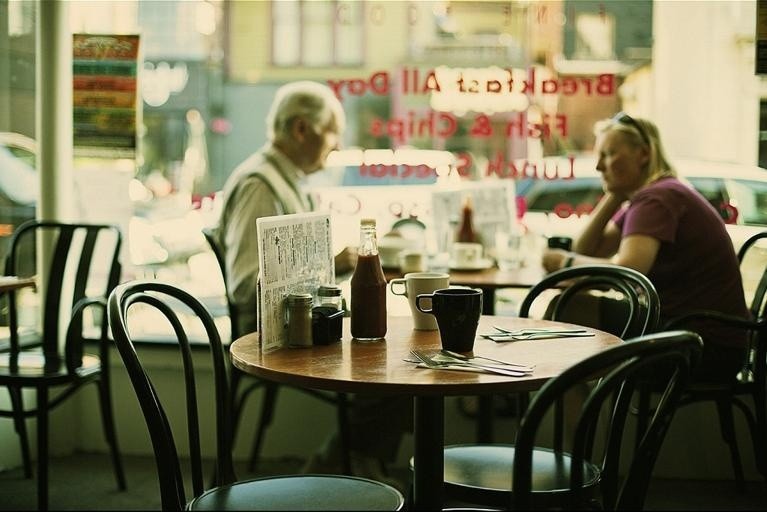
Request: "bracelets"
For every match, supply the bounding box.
[562,255,573,269]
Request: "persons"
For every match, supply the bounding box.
[540,112,753,390]
[215,80,413,498]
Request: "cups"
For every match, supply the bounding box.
[377,232,428,276]
[389,272,451,330]
[493,222,528,271]
[414,287,484,353]
[451,242,484,264]
[548,236,573,253]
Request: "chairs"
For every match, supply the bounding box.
[0,220,767,512]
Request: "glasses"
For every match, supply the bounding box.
[614,109,649,147]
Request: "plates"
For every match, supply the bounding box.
[446,259,495,272]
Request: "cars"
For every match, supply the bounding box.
[0,148,472,326]
[504,155,766,316]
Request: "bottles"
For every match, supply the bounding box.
[286,293,314,350]
[317,284,343,311]
[350,218,387,340]
[454,196,478,241]
